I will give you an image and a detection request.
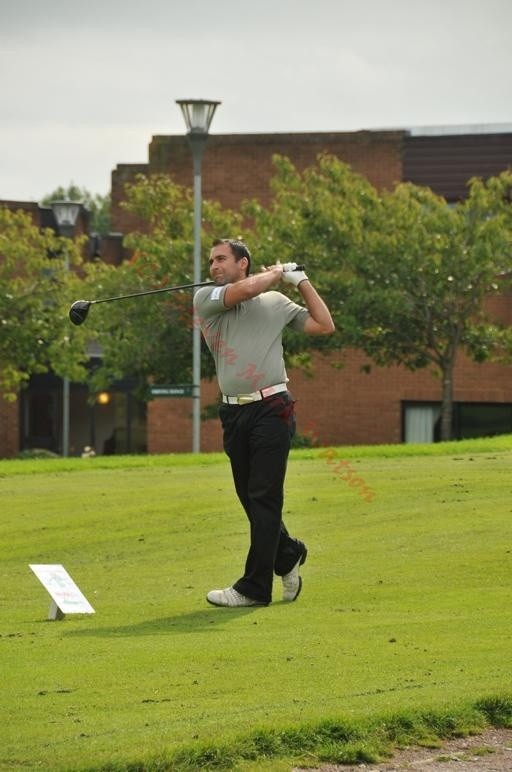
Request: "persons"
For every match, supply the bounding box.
[193,237,336,609]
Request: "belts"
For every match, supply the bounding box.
[222,383,287,405]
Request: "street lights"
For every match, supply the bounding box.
[177,99,223,457]
[50,200,84,459]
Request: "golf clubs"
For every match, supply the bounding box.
[68,263,306,326]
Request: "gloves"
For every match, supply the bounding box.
[281,262,308,287]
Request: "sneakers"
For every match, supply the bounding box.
[282,547,307,602]
[206,586,269,607]
